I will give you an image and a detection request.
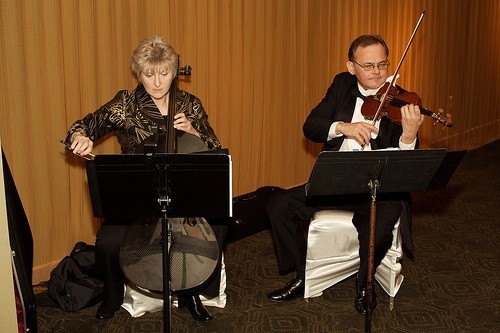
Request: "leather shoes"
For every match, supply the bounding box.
[268,278,304,302]
[355,274,376,315]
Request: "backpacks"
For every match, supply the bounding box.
[47,242,104,312]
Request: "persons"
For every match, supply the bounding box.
[265,35,425,314]
[68,35,227,322]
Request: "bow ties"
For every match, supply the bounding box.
[352,89,366,100]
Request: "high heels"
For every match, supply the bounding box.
[96,293,123,320]
[177,291,213,322]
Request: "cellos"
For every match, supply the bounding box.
[118,53,223,299]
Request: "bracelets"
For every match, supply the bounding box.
[70,131,84,143]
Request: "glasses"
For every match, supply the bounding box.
[353,59,390,71]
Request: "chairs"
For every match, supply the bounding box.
[303,209,403,312]
[121,248,227,318]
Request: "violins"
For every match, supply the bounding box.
[361,82,456,137]
